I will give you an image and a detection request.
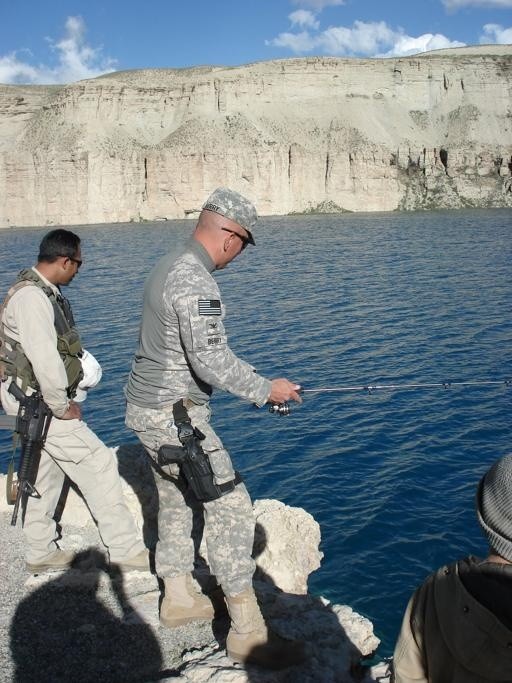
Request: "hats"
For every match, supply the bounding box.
[73,348,102,403]
[201,188,257,246]
[477,456,512,562]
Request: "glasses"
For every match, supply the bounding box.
[61,256,82,268]
[222,228,249,251]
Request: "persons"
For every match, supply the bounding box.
[1,229,155,573]
[123,186,319,670]
[389,452,511,683]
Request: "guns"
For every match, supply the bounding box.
[158,444,220,501]
[1,381,48,525]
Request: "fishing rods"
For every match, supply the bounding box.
[270,381,512,415]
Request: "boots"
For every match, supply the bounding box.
[26,550,76,573]
[159,572,228,628]
[224,588,305,670]
[110,548,155,572]
